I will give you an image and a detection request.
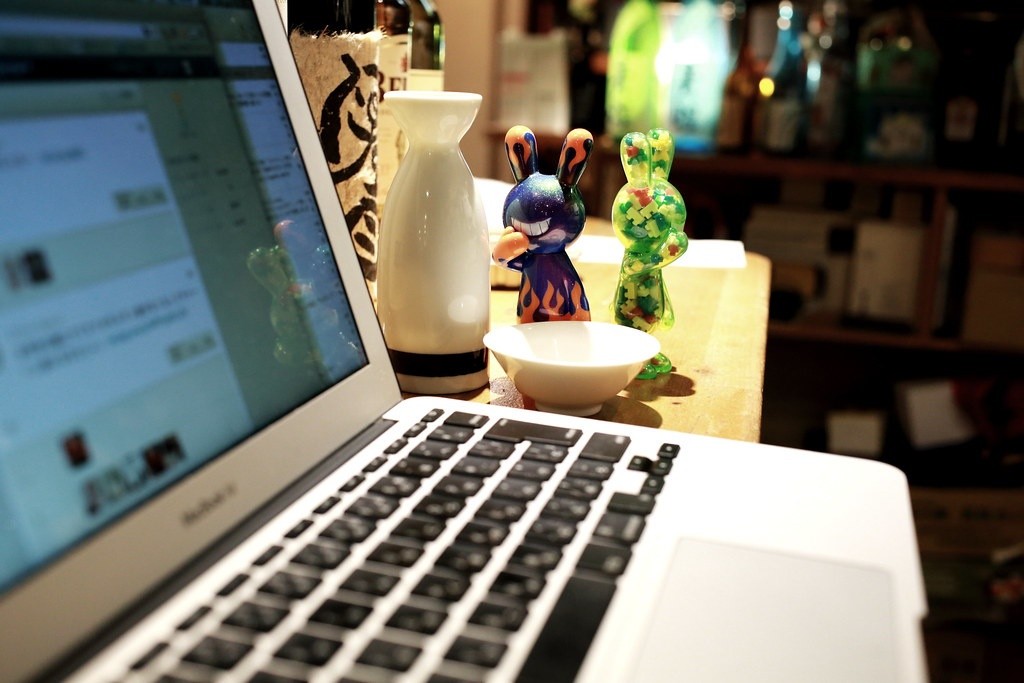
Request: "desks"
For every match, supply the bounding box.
[364,238,773,442]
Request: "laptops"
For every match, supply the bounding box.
[0,0,931,683]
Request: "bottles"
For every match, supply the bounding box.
[376,86,492,394]
[939,11,995,163]
[604,0,730,155]
[287,0,444,281]
[751,10,807,151]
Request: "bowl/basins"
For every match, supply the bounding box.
[482,320,661,418]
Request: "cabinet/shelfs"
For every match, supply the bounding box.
[494,128,1024,580]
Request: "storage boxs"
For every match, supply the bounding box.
[742,203,924,325]
[961,229,1023,347]
